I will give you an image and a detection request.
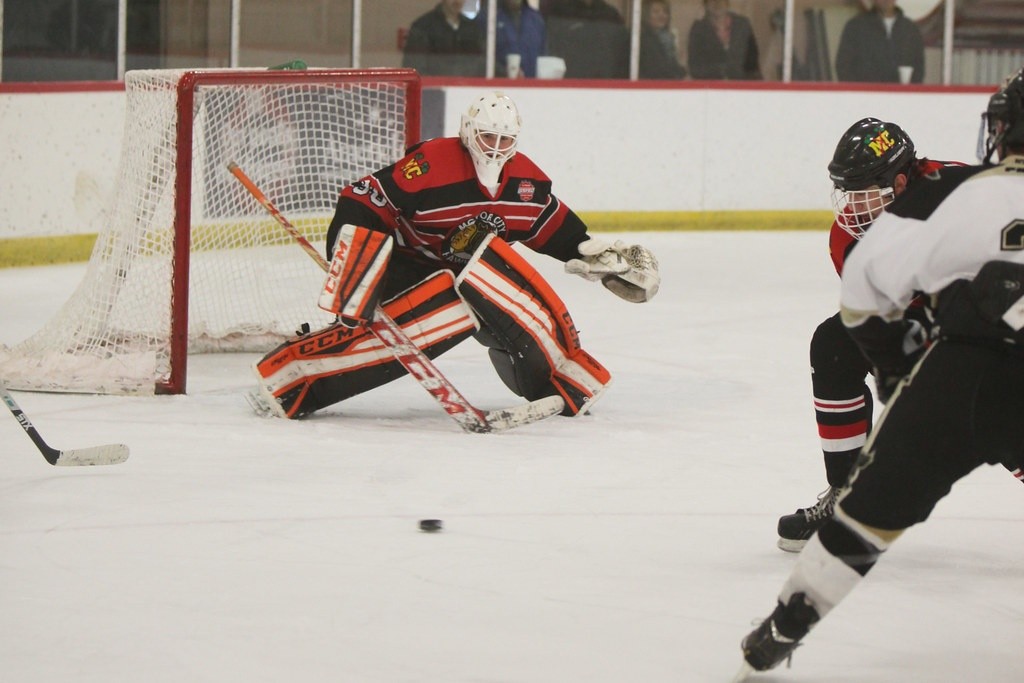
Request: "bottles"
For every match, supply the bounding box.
[267,60,307,70]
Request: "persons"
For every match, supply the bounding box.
[731,65,1024,683]
[401,0,926,85]
[325,93,630,418]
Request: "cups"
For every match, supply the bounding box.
[898,66,914,84]
[507,54,521,79]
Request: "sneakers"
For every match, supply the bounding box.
[735,584,820,682]
[777,484,850,553]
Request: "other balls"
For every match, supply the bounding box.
[418,520,443,531]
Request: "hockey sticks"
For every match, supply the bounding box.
[226,156,566,437]
[0,381,130,466]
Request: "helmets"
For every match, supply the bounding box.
[827,117,915,240]
[458,92,521,188]
[987,67,1024,143]
